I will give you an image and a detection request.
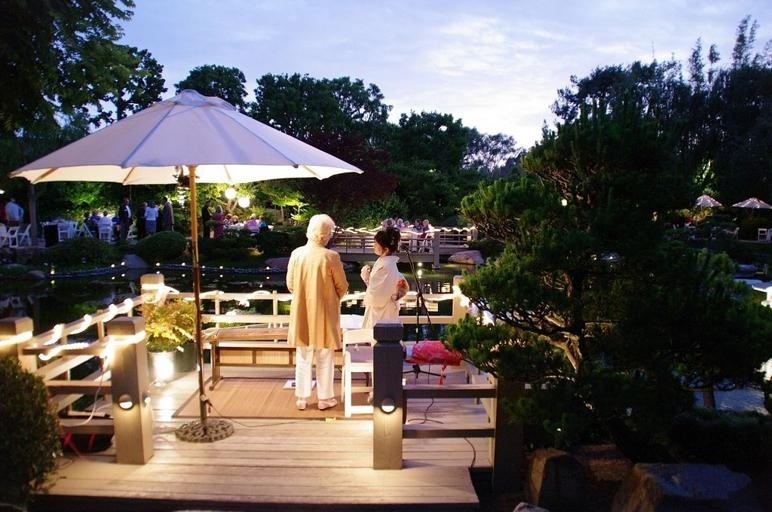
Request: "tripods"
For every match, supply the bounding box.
[402,297,447,381]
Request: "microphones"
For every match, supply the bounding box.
[400,243,413,254]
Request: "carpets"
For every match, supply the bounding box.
[170,373,376,422]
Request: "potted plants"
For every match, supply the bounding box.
[137,296,198,381]
[174,298,205,375]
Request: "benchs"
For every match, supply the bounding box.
[202,324,343,391]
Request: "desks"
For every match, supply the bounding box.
[223,221,274,236]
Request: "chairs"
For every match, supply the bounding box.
[339,329,404,416]
[381,224,437,253]
[0,213,137,250]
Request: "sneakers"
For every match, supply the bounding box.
[296,400,306,410]
[318,399,337,409]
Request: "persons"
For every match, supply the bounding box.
[361,228,409,406]
[83,195,175,240]
[212,205,226,239]
[5,196,24,247]
[380,216,434,252]
[285,214,351,410]
[201,200,212,239]
[223,210,268,236]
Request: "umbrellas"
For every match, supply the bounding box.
[7,87,365,444]
